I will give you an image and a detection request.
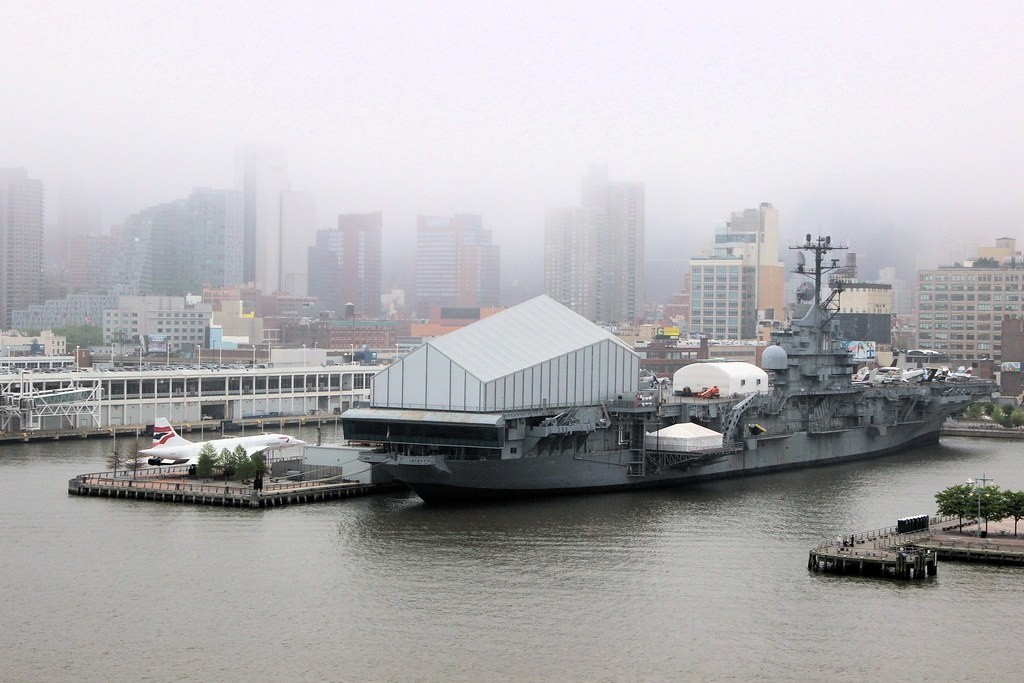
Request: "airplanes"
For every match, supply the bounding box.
[138,417,308,467]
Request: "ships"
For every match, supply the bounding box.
[339,224,997,499]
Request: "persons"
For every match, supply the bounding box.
[851,535,855,547]
[711,386,720,399]
[857,342,866,359]
[866,343,875,359]
